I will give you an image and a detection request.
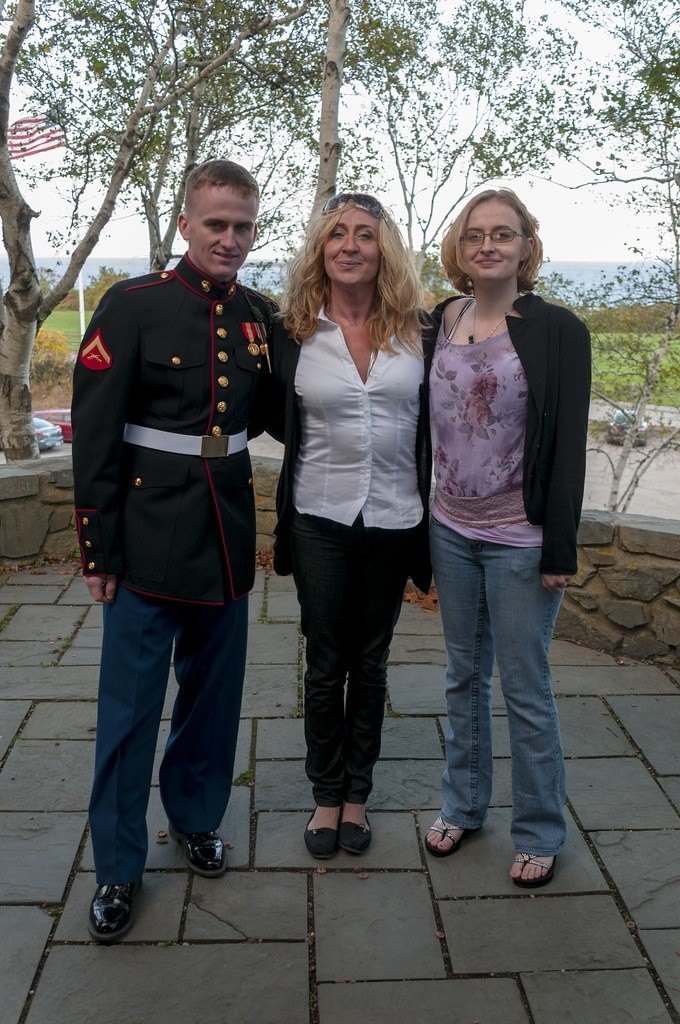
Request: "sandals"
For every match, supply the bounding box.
[513,852,557,887]
[425,816,482,858]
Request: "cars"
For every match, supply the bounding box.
[32,410,73,442]
[608,410,649,447]
[33,418,63,450]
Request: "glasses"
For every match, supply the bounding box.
[320,194,391,229]
[460,228,524,246]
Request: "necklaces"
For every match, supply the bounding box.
[468,304,515,348]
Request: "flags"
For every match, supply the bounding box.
[7,102,67,159]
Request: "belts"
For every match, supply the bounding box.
[123,423,251,458]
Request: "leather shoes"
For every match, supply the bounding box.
[88,880,143,943]
[169,823,227,876]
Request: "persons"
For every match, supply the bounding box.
[425,190,591,888]
[70,158,284,940]
[271,194,433,859]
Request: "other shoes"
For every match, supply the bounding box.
[339,814,372,853]
[304,804,343,858]
